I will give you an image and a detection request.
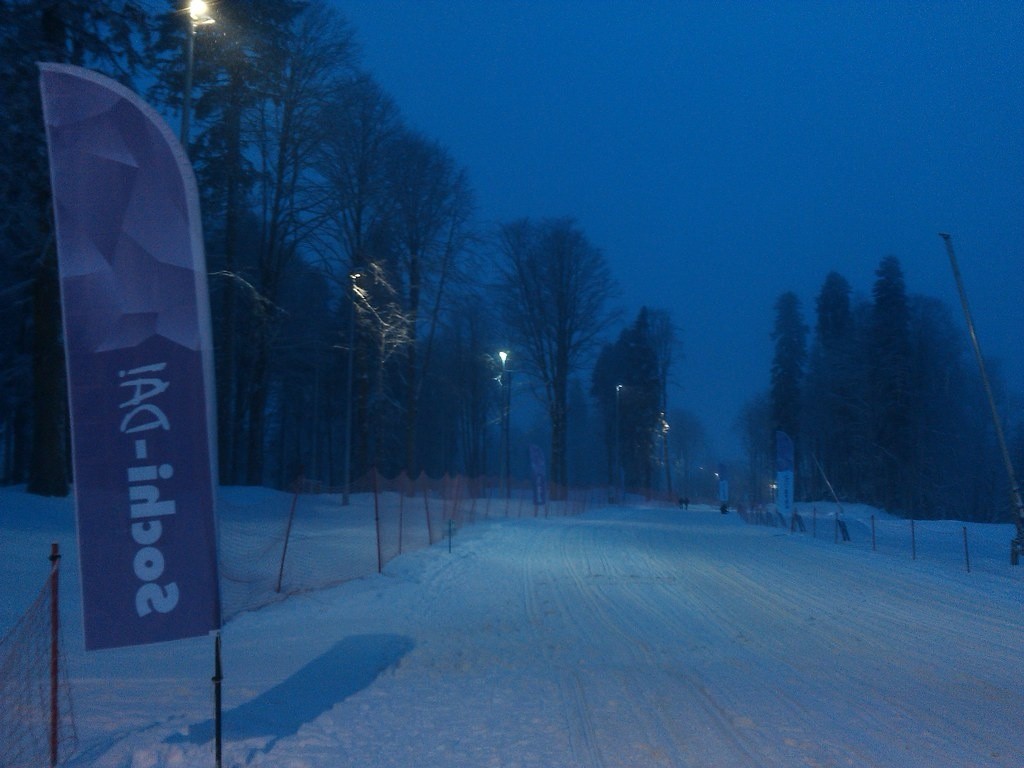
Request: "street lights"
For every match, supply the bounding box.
[497,349,512,499]
[181,0,210,154]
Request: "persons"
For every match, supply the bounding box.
[720,504,729,514]
[679,498,688,510]
